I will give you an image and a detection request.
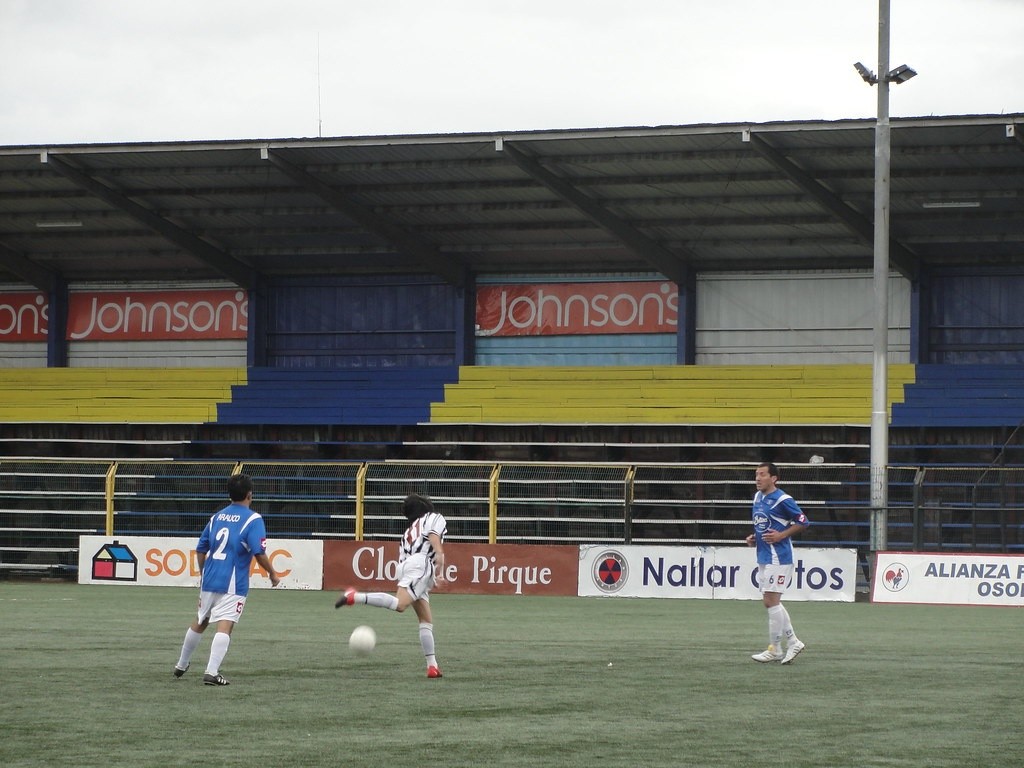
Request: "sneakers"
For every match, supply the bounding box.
[203,671,231,687]
[782,641,806,665]
[335,587,356,609]
[751,648,783,663]
[173,661,191,677]
[426,664,443,678]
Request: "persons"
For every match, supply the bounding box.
[173,475,279,686]
[335,491,448,678]
[746,464,809,663]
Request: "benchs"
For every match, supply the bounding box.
[0,363,1024,425]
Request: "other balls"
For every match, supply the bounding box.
[350,626,376,655]
